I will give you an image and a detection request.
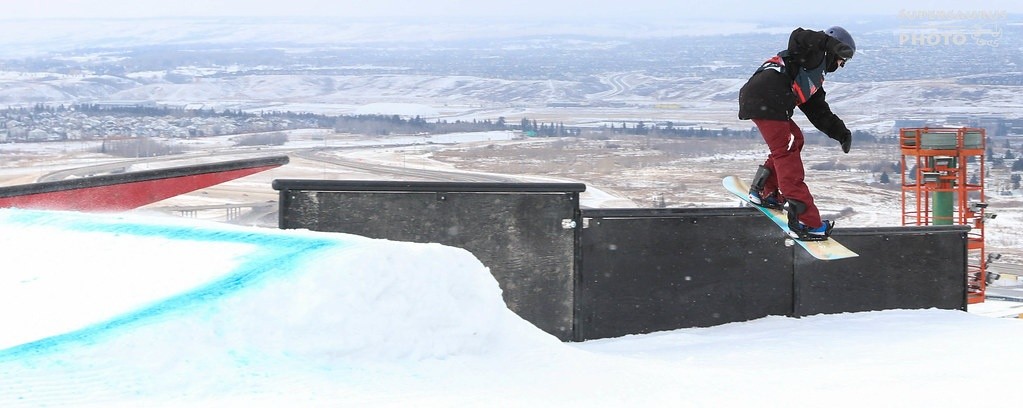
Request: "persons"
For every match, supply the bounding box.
[737,25,854,243]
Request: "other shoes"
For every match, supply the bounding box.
[748,165,787,211]
[777,197,835,241]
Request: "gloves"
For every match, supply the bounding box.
[827,114,852,153]
[785,54,800,81]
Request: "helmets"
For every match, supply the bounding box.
[823,25,856,53]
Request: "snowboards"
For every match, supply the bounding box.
[720,174,861,261]
